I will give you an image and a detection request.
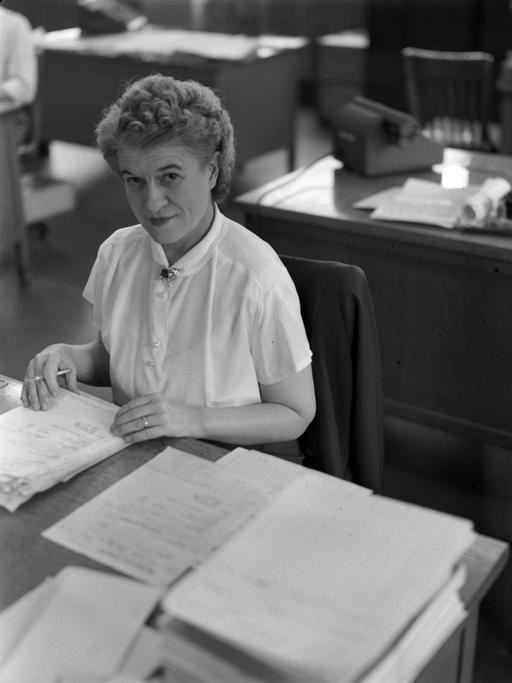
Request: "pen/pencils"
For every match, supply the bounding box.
[26,369,72,382]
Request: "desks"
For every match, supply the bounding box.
[233,135,510,454]
[0,369,511,682]
[30,19,311,177]
[0,105,34,290]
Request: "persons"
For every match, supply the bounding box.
[19,75,317,464]
[0,0,39,146]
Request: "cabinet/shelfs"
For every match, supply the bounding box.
[316,29,377,126]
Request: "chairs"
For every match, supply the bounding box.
[274,252,385,493]
[398,44,495,150]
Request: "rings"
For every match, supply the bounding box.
[140,416,149,427]
